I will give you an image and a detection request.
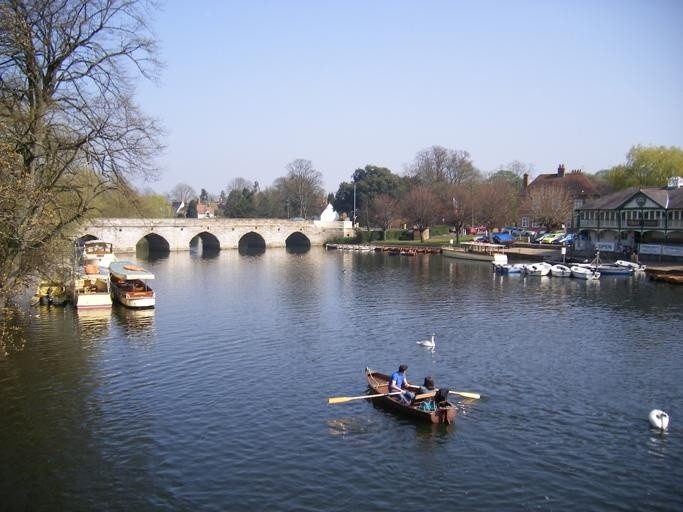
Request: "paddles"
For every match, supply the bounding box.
[409,385,480,399]
[328,392,402,404]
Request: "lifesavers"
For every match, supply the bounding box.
[85,264,100,274]
[123,264,143,271]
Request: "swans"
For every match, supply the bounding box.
[648,409,670,430]
[416,333,435,346]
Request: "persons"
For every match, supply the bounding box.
[410,372,435,412]
[389,364,414,405]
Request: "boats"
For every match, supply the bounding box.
[365,366,456,424]
[497,259,646,279]
[108,261,156,308]
[81,239,116,267]
[71,277,113,309]
[29,280,67,320]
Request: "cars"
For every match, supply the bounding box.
[502,225,537,236]
[464,227,476,235]
[530,233,574,245]
